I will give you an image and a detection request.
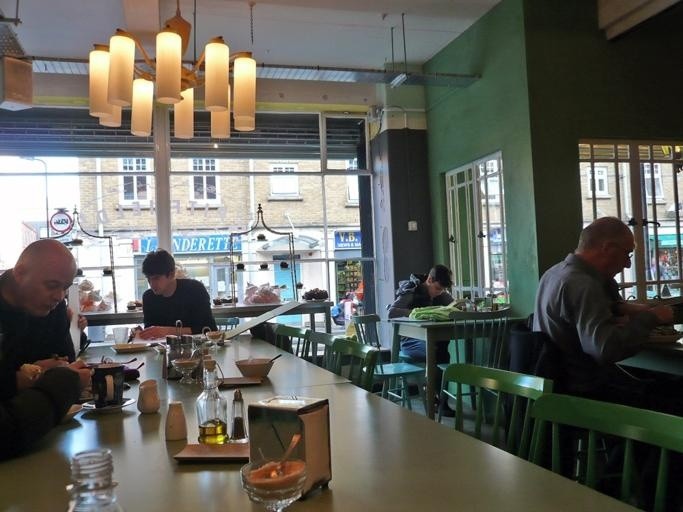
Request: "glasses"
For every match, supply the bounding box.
[104,357,144,369]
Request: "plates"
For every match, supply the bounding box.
[112,343,152,353]
[173,443,248,460]
[81,398,137,414]
[647,331,682,344]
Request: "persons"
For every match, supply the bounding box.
[0,238,83,391]
[133,247,217,341]
[385,263,458,420]
[529,214,663,482]
[1,358,97,462]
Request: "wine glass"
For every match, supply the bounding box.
[206,331,221,357]
[240,458,308,512]
[171,358,201,385]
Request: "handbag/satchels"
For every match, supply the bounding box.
[409,305,463,321]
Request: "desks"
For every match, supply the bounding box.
[79,302,334,365]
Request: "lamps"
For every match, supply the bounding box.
[390,13,408,89]
[90,0,258,139]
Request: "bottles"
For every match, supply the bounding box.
[193,360,228,437]
[64,448,124,512]
[135,379,160,414]
[164,334,205,383]
[164,402,187,442]
[229,390,248,444]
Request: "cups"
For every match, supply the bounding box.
[89,363,124,408]
[113,327,128,345]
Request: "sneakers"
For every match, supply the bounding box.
[422,384,455,417]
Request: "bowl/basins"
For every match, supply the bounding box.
[235,359,274,382]
